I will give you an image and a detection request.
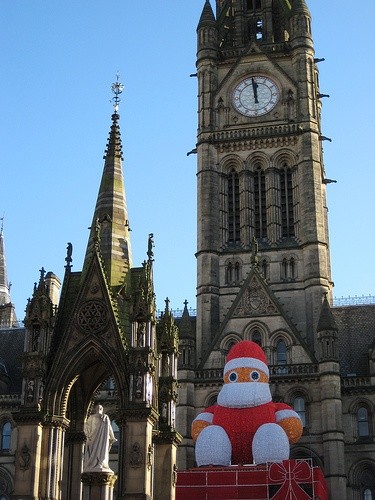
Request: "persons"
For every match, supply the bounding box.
[82,405,116,472]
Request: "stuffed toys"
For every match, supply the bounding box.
[191,340,304,467]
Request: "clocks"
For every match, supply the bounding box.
[232,74,281,118]
